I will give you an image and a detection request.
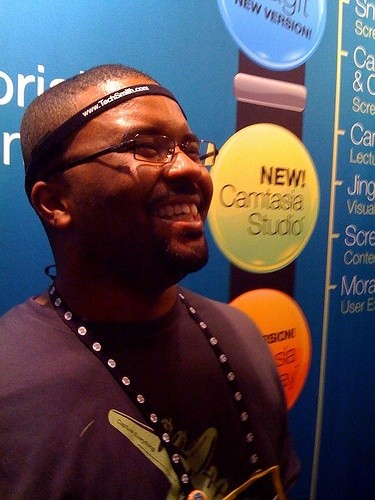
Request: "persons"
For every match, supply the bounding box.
[1,62,315,500]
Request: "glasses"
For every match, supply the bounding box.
[42,132,219,186]
